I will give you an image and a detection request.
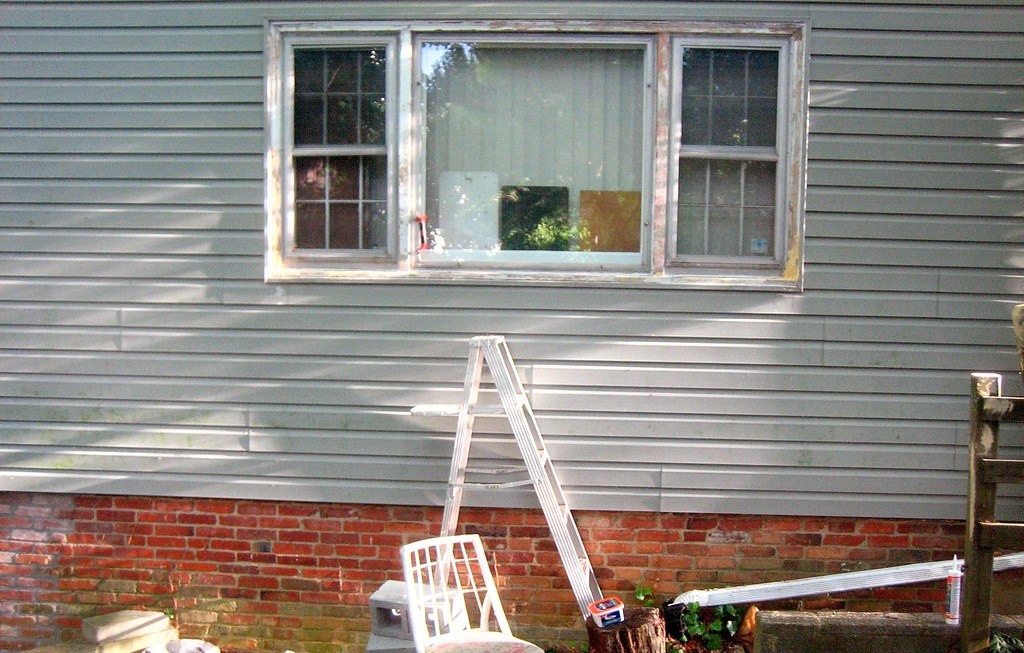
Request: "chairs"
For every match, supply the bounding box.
[399,534,545,653]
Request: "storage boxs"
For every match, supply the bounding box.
[587,597,625,627]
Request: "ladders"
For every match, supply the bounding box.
[430,335,608,619]
